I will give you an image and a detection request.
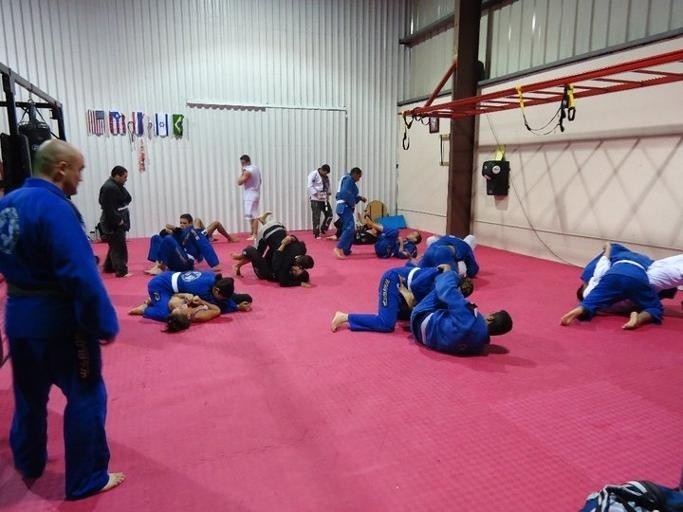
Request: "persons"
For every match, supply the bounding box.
[129,214,314,333]
[1,137,126,494]
[307,163,333,240]
[359,213,421,260]
[330,236,514,357]
[331,167,367,257]
[238,154,273,239]
[560,243,682,331]
[99,161,133,277]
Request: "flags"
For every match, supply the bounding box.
[87,109,103,136]
[154,112,168,137]
[108,111,126,136]
[172,115,184,139]
[128,111,150,170]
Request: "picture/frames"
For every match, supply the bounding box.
[428,115,439,134]
